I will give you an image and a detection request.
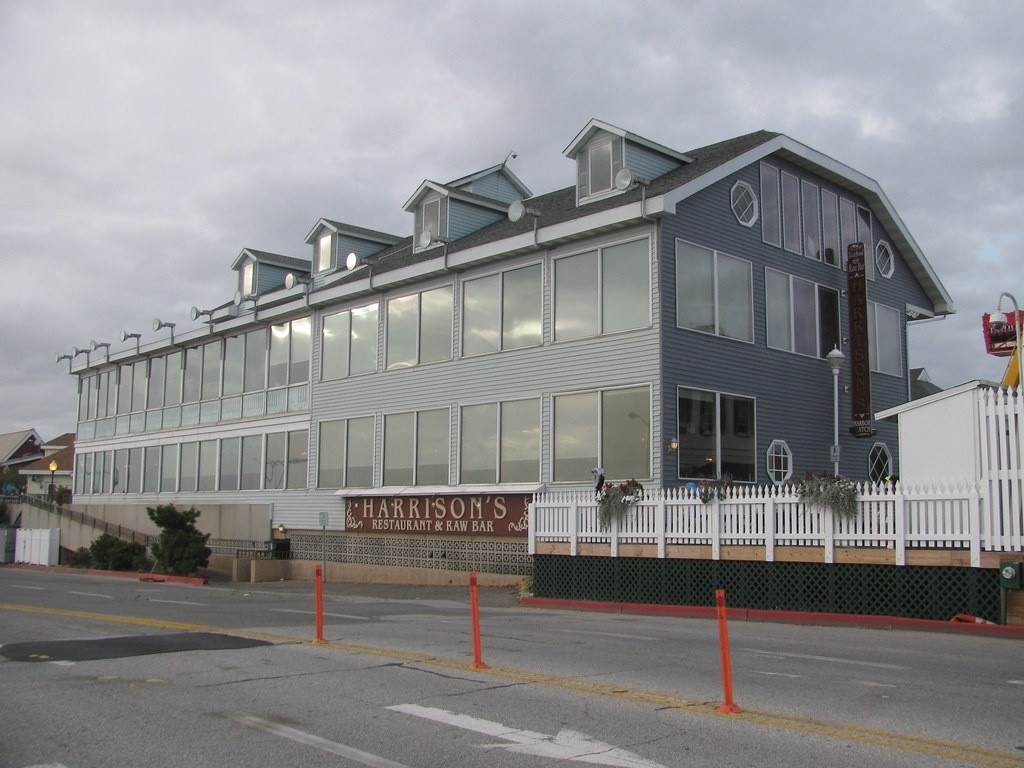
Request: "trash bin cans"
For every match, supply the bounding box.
[14,527,61,567]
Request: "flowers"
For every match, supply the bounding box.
[798,469,859,519]
[698,471,736,504]
[596,478,647,535]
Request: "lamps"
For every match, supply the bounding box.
[153,318,182,349]
[285,274,324,311]
[615,168,658,222]
[509,200,552,250]
[71,346,99,370]
[190,306,225,337]
[55,353,80,375]
[234,291,270,325]
[120,330,149,357]
[667,437,678,455]
[419,231,462,274]
[347,253,385,293]
[89,340,119,365]
[278,524,286,534]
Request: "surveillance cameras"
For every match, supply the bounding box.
[592,467,605,476]
[990,314,1007,331]
